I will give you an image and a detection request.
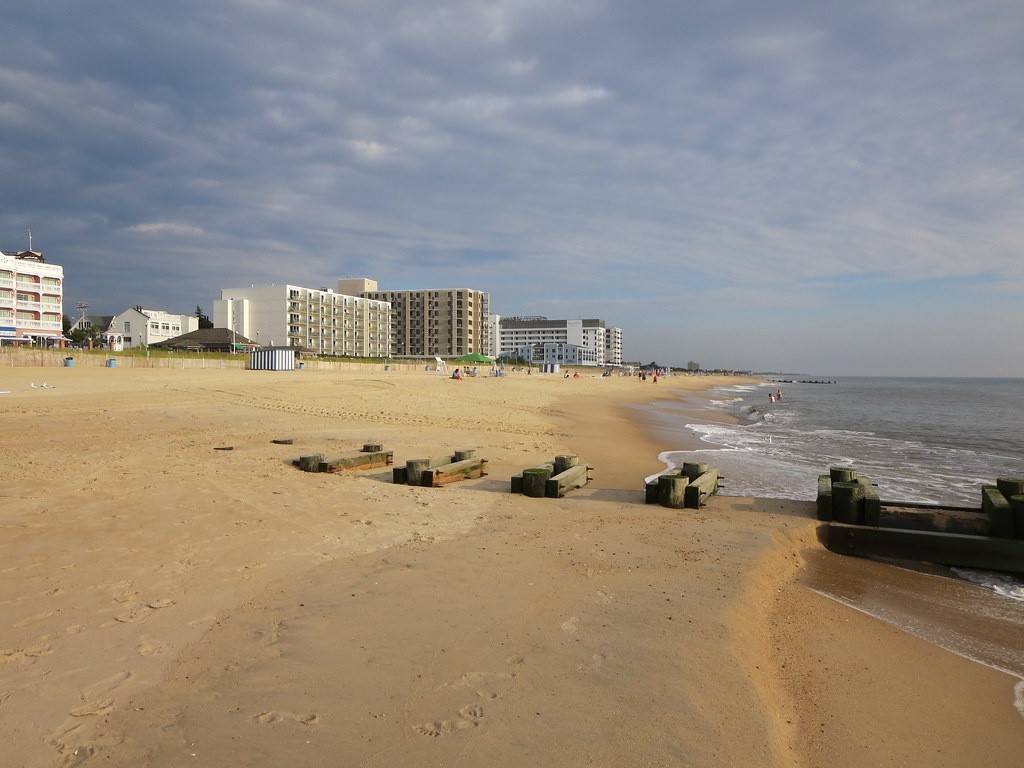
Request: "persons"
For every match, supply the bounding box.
[452,369,461,379]
[652,376,657,383]
[776,390,783,398]
[639,371,642,381]
[642,374,646,380]
[564,370,569,378]
[574,371,580,377]
[463,366,479,377]
[769,394,775,402]
